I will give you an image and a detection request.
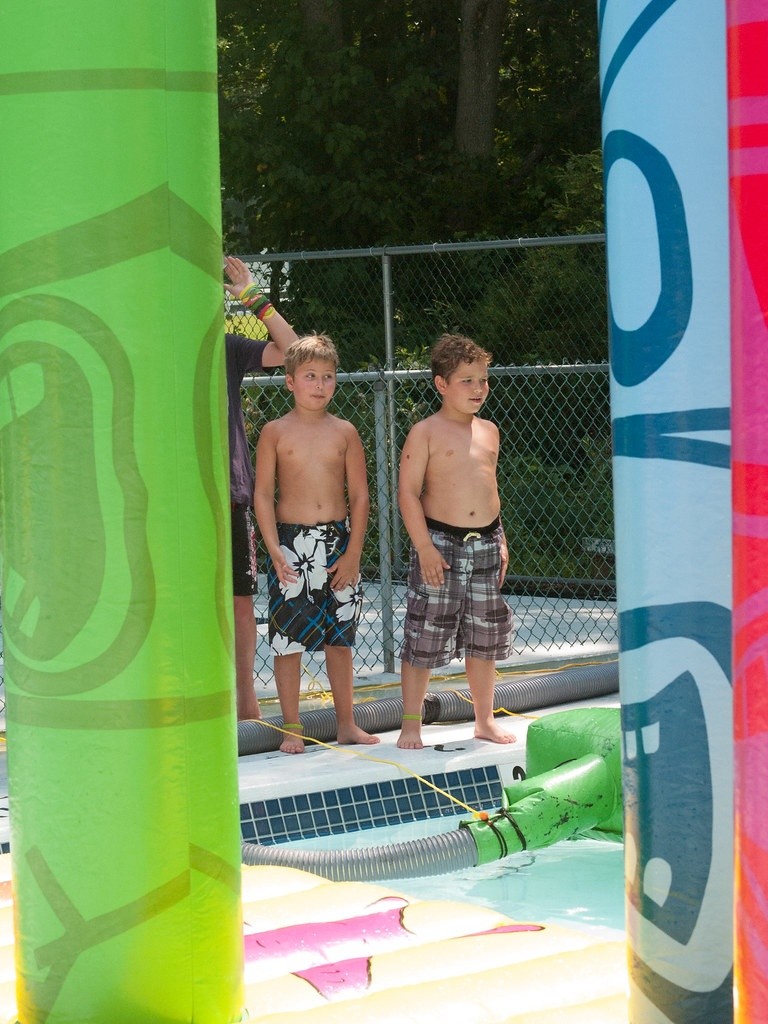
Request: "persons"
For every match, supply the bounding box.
[254,335,380,753]
[223,256,300,721]
[396,333,518,749]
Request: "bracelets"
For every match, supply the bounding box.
[239,283,275,321]
